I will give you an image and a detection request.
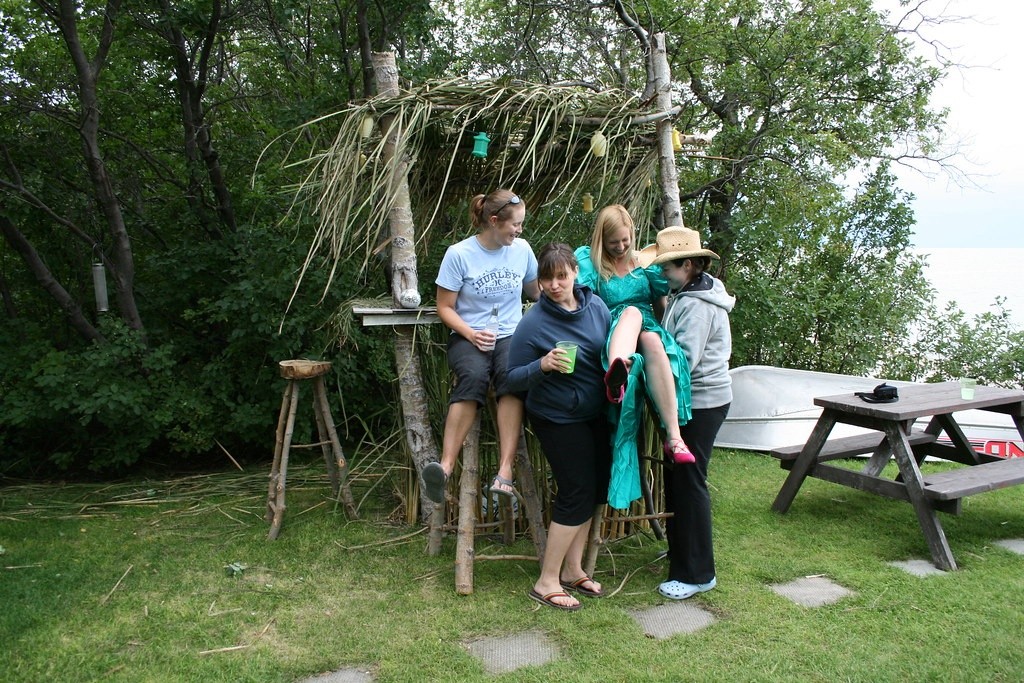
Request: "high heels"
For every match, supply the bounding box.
[663,436,695,464]
[605,357,629,408]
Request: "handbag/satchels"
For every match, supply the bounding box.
[855,384,900,403]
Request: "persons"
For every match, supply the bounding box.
[623,225,734,602]
[420,189,543,504]
[506,242,636,610]
[573,204,714,465]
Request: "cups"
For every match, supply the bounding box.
[960,377,976,400]
[555,340,578,374]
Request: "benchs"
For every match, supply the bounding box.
[771,427,934,475]
[922,456,1023,516]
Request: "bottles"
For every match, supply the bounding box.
[582,193,595,212]
[481,308,499,351]
[590,131,607,157]
[672,129,682,151]
[358,111,374,138]
[471,132,491,157]
[359,153,366,174]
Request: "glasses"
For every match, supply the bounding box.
[494,194,522,216]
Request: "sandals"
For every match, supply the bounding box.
[658,574,717,599]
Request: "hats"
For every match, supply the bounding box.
[637,225,720,270]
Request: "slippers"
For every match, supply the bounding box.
[422,461,448,503]
[529,587,583,610]
[559,576,603,596]
[489,475,515,496]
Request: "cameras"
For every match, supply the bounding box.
[874,383,898,400]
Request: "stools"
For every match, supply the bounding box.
[264,359,357,542]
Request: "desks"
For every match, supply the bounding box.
[770,382,1024,572]
